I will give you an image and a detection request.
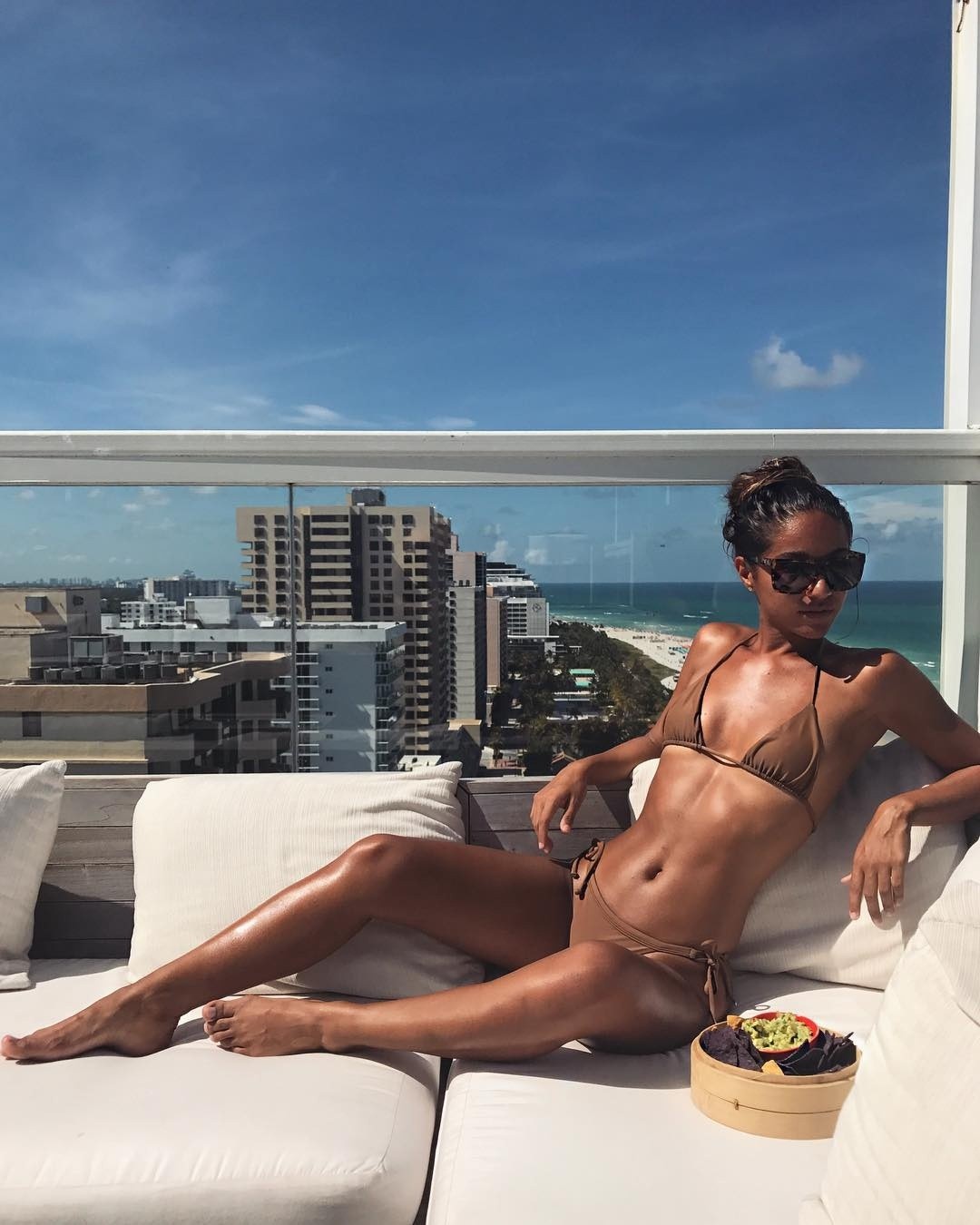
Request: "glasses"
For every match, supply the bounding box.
[746,550,866,594]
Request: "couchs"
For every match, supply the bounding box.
[2,956,892,1224]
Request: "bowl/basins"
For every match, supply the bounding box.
[751,1011,819,1060]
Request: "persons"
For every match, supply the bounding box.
[0,456,980,1066]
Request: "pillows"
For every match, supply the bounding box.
[820,839,979,1225]
[0,756,61,986]
[130,763,487,1006]
[623,734,970,991]
